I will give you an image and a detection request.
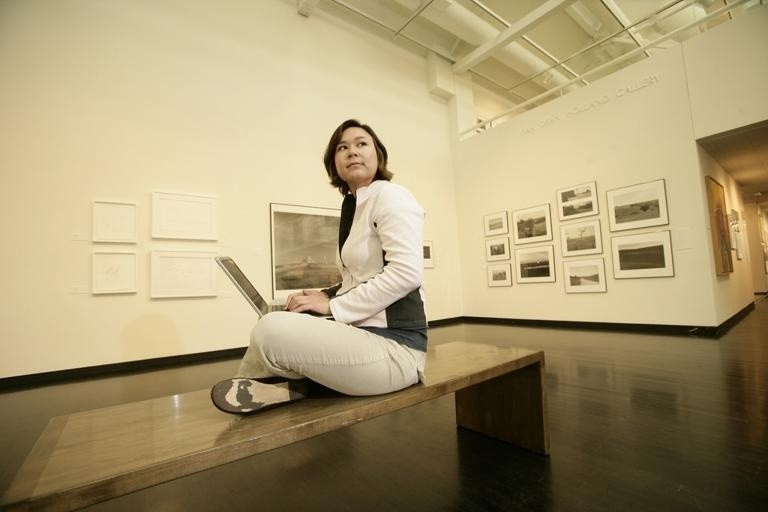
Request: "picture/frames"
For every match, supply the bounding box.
[705,175,734,277]
[482,179,675,294]
[270,202,343,299]
[91,190,219,300]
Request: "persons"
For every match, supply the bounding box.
[209,116,431,417]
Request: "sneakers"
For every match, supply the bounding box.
[211,379,307,416]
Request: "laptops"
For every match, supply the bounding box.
[214,255,340,324]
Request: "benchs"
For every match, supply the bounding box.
[1,341,554,511]
